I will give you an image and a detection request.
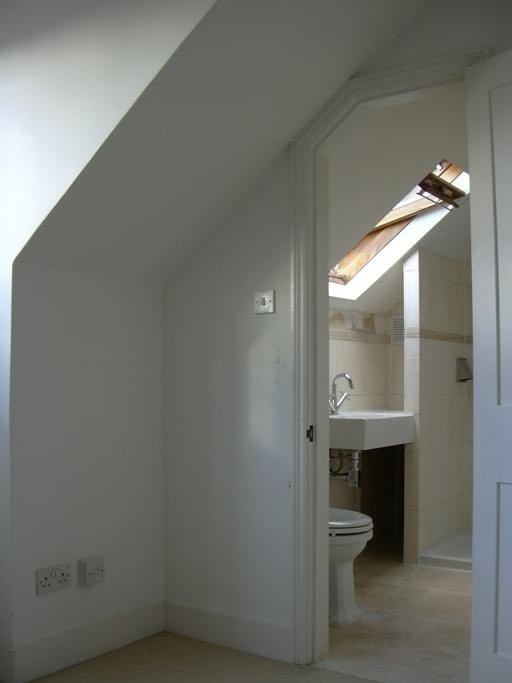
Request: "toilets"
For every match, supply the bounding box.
[328,506,375,630]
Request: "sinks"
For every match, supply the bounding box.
[327,411,417,451]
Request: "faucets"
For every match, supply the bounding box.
[331,372,355,413]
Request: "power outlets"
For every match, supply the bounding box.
[36,556,105,595]
